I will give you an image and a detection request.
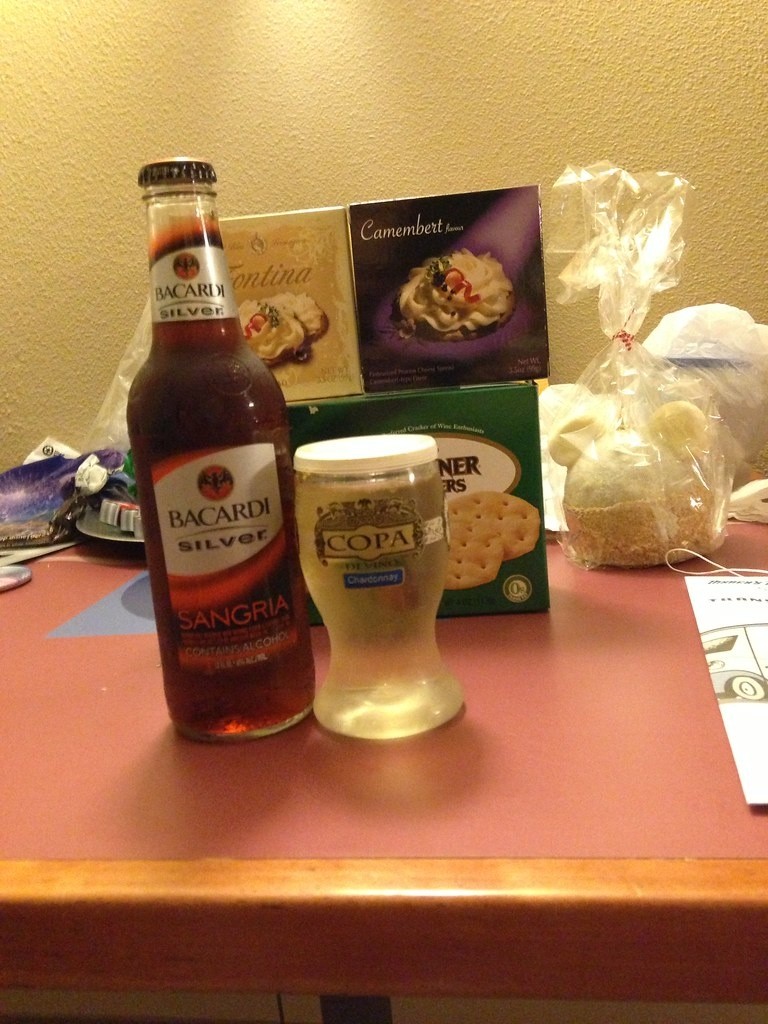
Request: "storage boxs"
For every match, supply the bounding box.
[352,183,551,393]
[221,205,363,403]
[285,379,549,628]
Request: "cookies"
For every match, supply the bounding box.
[447,492,540,558]
[443,520,504,590]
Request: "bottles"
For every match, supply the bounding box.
[127,160,315,743]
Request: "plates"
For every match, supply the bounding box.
[75,507,147,541]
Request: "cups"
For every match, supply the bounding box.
[294,434,464,739]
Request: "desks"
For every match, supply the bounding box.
[0,524,768,1024]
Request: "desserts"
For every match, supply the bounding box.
[396,249,514,339]
[235,291,328,365]
